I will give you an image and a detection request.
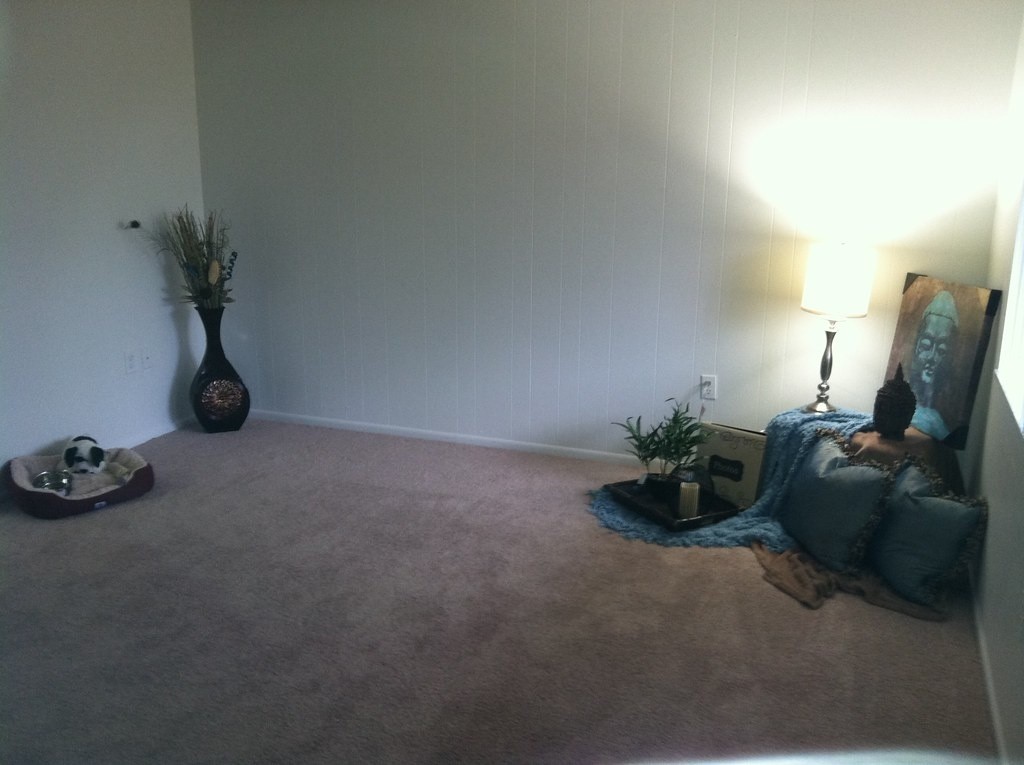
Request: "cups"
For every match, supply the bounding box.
[679,482,700,518]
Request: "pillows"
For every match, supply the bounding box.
[867,450,988,601]
[777,429,901,578]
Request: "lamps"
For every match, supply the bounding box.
[799,236,876,410]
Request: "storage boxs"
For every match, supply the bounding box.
[696,422,768,511]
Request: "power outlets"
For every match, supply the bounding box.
[701,374,717,400]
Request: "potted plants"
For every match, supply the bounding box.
[137,200,252,433]
[615,396,716,496]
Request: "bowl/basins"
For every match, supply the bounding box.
[34,470,73,496]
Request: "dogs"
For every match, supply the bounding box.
[57,434,105,475]
